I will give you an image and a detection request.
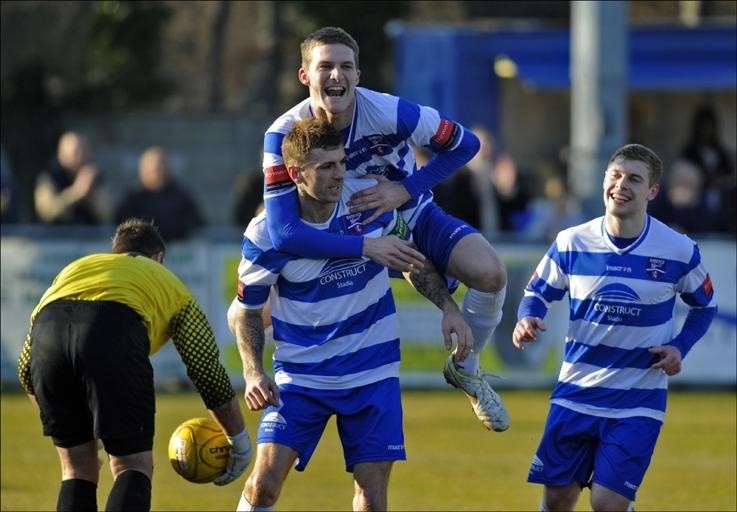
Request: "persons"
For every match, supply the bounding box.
[234,148,263,224]
[655,158,716,238]
[34,130,111,224]
[226,25,512,432]
[109,144,208,243]
[235,116,475,512]
[430,129,587,243]
[512,143,718,512]
[18,217,253,511]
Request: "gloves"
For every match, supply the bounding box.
[211,429,252,487]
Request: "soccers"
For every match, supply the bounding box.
[168,416,230,485]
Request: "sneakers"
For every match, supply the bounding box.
[443,347,511,432]
